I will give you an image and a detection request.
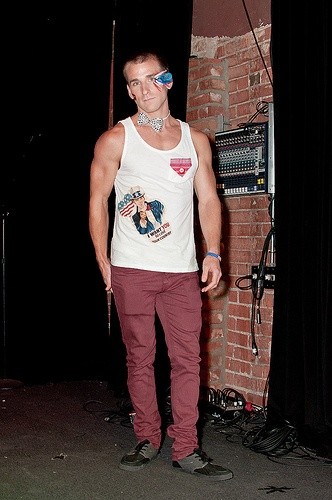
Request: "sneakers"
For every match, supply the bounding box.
[120,439,160,472]
[173,449,235,480]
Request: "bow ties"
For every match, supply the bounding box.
[137,112,164,134]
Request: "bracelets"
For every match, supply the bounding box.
[206,252,222,261]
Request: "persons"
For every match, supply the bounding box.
[89,53,234,481]
